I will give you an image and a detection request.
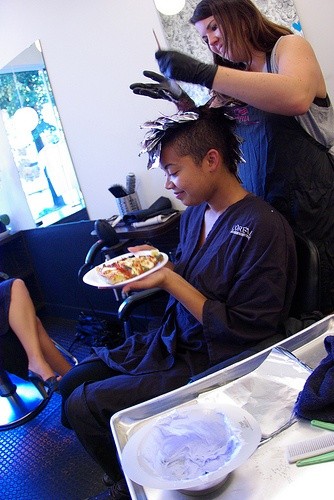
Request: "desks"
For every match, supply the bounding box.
[91,210,185,240]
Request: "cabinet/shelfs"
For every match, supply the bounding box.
[0,230,46,311]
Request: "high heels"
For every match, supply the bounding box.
[28,369,62,399]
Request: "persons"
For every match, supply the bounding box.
[0,278,73,388]
[56,107,299,500]
[130,1,334,322]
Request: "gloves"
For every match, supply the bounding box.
[129,70,195,111]
[155,51,219,89]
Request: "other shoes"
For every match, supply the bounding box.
[84,483,131,500]
[102,472,117,486]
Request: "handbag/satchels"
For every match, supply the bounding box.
[67,300,120,352]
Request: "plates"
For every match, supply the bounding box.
[82,249,169,288]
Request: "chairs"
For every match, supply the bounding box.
[118,232,321,384]
[0,272,78,430]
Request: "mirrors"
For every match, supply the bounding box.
[152,0,305,107]
[0,39,86,229]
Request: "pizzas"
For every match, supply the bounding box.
[96,255,164,284]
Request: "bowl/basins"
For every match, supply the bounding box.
[120,402,262,497]
